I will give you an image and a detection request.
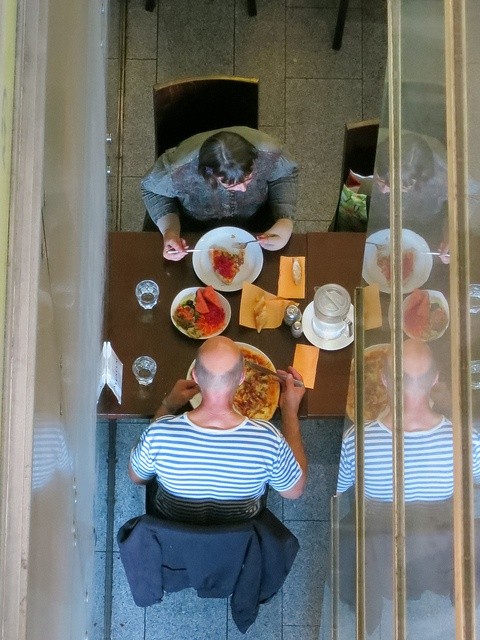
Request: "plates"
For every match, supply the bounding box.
[191,226,265,294]
[170,286,232,340]
[364,227,434,294]
[185,341,281,427]
[400,288,450,343]
[304,296,355,352]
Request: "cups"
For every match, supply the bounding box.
[468,360,480,391]
[136,280,160,309]
[132,355,158,387]
[466,283,480,313]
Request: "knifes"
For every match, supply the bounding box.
[244,358,304,388]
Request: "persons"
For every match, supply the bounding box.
[336,337,480,501]
[368,127,480,266]
[141,126,300,262]
[128,336,308,524]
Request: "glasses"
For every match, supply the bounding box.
[375,171,416,193]
[212,160,258,191]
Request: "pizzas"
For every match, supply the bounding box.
[376,246,416,291]
[210,246,244,285]
[232,344,281,421]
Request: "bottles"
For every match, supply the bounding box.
[291,322,302,338]
[283,302,303,326]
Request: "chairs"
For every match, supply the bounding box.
[329,120,380,232]
[116,424,300,633]
[340,516,479,634]
[143,74,261,230]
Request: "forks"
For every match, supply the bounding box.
[232,239,263,249]
[365,239,390,250]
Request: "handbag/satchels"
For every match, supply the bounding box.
[335,169,373,232]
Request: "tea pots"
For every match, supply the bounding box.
[312,282,354,339]
